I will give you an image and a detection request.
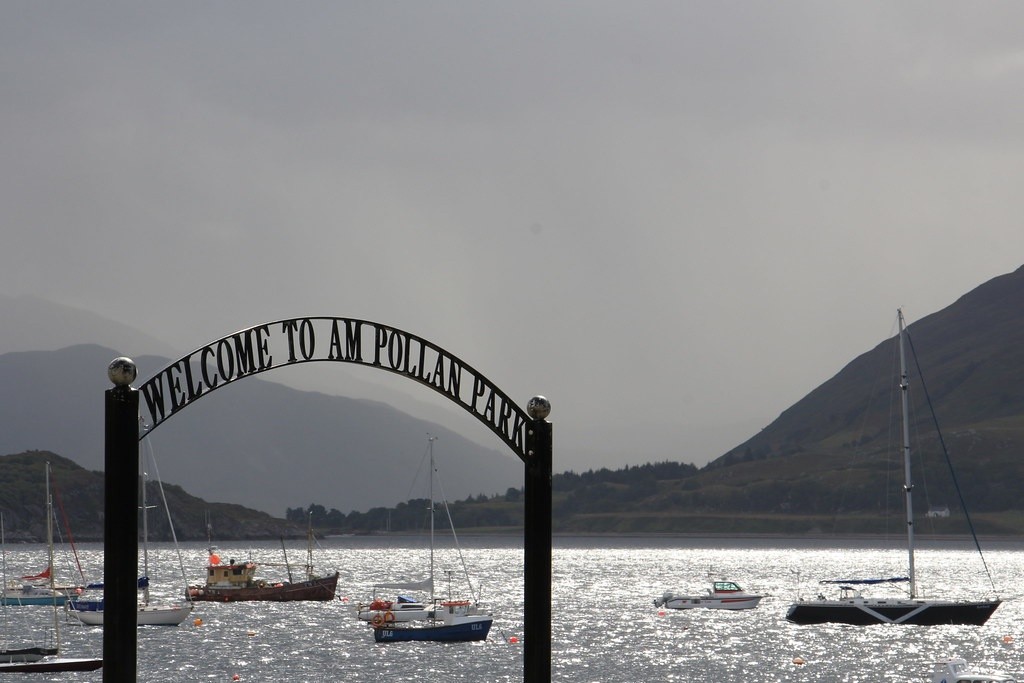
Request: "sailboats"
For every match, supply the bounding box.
[357,432,493,643]
[1,494,105,673]
[1,460,85,606]
[784,309,1004,627]
[62,407,195,626]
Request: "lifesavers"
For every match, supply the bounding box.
[259,581,264,588]
[384,612,394,623]
[372,615,384,627]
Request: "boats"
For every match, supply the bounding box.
[184,509,340,602]
[651,580,764,610]
